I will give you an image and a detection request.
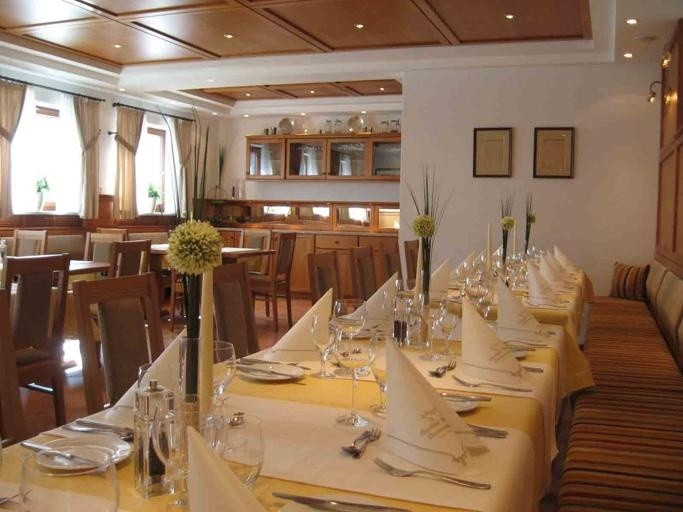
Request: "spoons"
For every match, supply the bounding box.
[339,429,370,454]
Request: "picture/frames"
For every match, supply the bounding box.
[532,125,573,180]
[472,126,512,178]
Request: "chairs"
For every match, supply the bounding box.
[1,221,423,441]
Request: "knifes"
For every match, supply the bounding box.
[76,417,131,432]
[270,488,410,512]
[17,438,96,466]
[236,364,299,380]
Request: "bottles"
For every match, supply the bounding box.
[132,378,173,501]
[237,179,245,201]
[0,239,6,264]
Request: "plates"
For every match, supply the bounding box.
[36,432,129,470]
[346,116,363,133]
[278,117,294,135]
[235,361,304,385]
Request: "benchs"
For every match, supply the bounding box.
[549,254,681,512]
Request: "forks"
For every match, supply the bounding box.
[60,422,132,440]
[0,487,19,507]
[353,427,384,461]
[371,457,492,492]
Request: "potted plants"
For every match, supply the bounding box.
[402,164,457,320]
[146,183,161,212]
[32,175,51,210]
[152,104,222,432]
[523,192,537,253]
[490,190,515,284]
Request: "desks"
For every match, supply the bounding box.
[0,306,588,512]
[436,266,590,346]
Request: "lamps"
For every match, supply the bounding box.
[660,36,675,67]
[644,79,670,105]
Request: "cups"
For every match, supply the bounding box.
[18,447,120,512]
[334,119,345,135]
[389,119,399,133]
[270,126,276,135]
[136,362,152,384]
[324,120,332,134]
[263,128,269,136]
[378,120,388,134]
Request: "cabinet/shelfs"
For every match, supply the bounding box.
[326,135,399,184]
[244,132,328,180]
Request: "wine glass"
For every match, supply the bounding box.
[152,336,266,511]
[310,242,545,426]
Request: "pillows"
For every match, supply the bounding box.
[608,256,648,305]
